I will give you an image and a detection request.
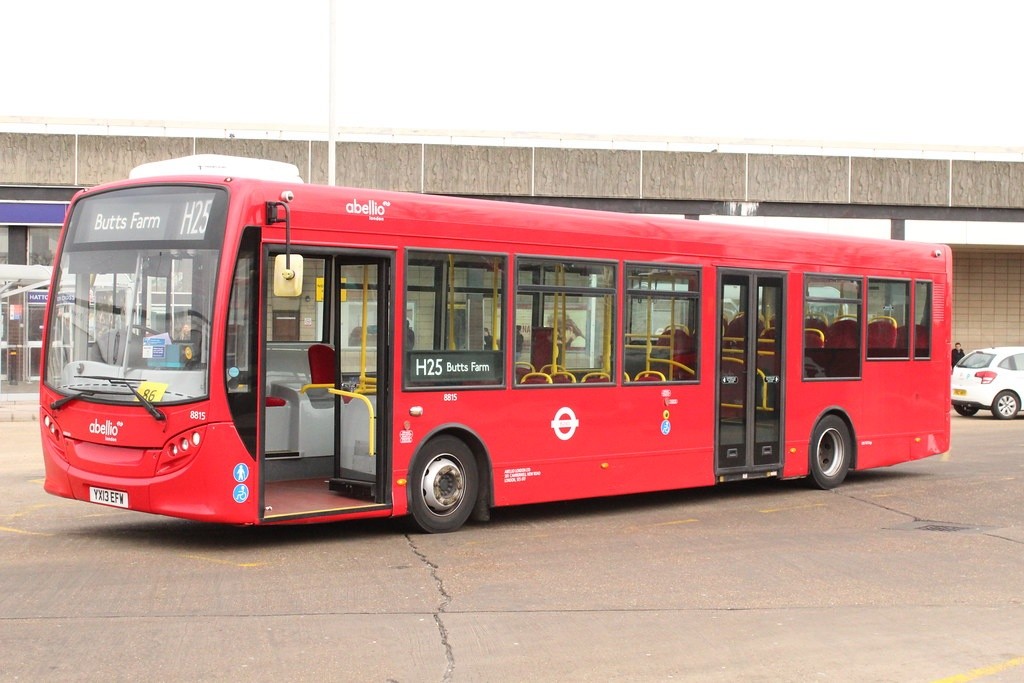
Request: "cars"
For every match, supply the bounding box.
[951,346,1024,420]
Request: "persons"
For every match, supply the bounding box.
[951,342,966,370]
[406,320,414,351]
[484,327,492,349]
[549,312,586,347]
[515,325,524,362]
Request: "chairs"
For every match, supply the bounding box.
[308,344,339,384]
[515,314,928,385]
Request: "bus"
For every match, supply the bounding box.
[39,156,952,535]
